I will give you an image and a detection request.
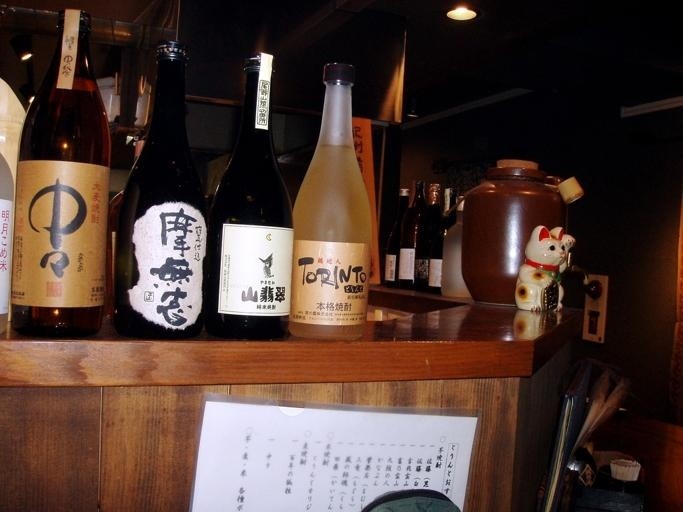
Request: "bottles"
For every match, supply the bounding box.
[115,41,211,345]
[385,160,568,306]
[11,1,106,343]
[288,64,373,342]
[210,51,295,345]
[0,151,13,339]
